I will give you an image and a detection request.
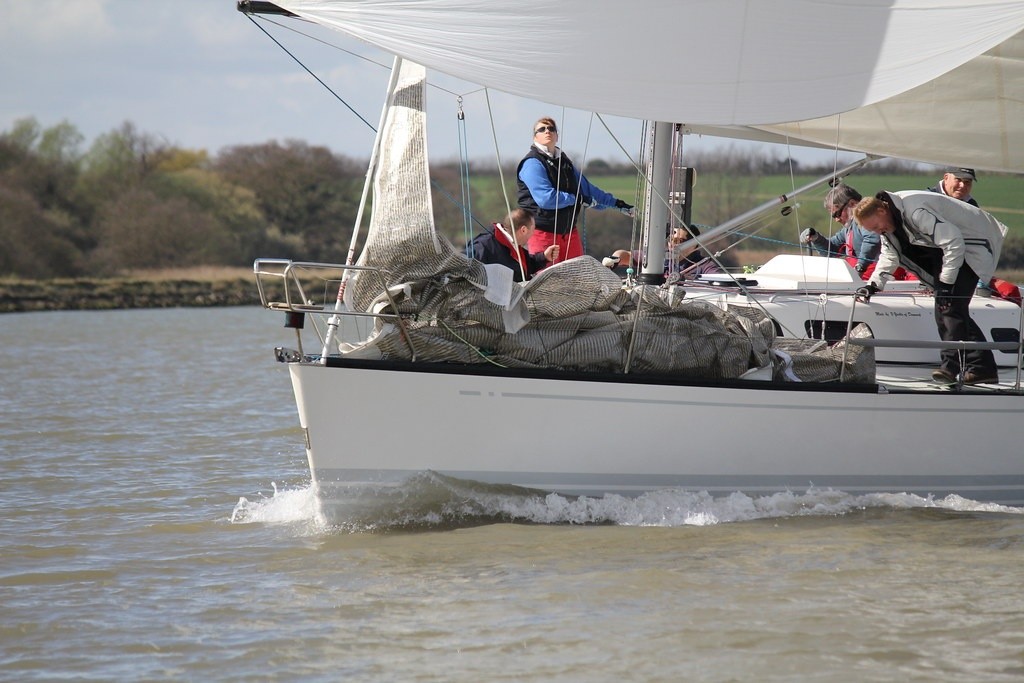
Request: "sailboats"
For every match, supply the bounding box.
[238,0,1024,527]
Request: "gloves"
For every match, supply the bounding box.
[615,199,635,217]
[580,196,597,209]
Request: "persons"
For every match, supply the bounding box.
[925,167,1022,307]
[461,209,560,282]
[800,182,920,282]
[852,189,1009,385]
[602,225,731,286]
[517,117,635,275]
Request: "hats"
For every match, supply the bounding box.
[946,167,977,183]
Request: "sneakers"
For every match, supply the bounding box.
[932,370,958,389]
[963,371,998,384]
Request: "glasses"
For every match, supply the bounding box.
[673,237,687,244]
[832,198,856,218]
[535,126,556,137]
